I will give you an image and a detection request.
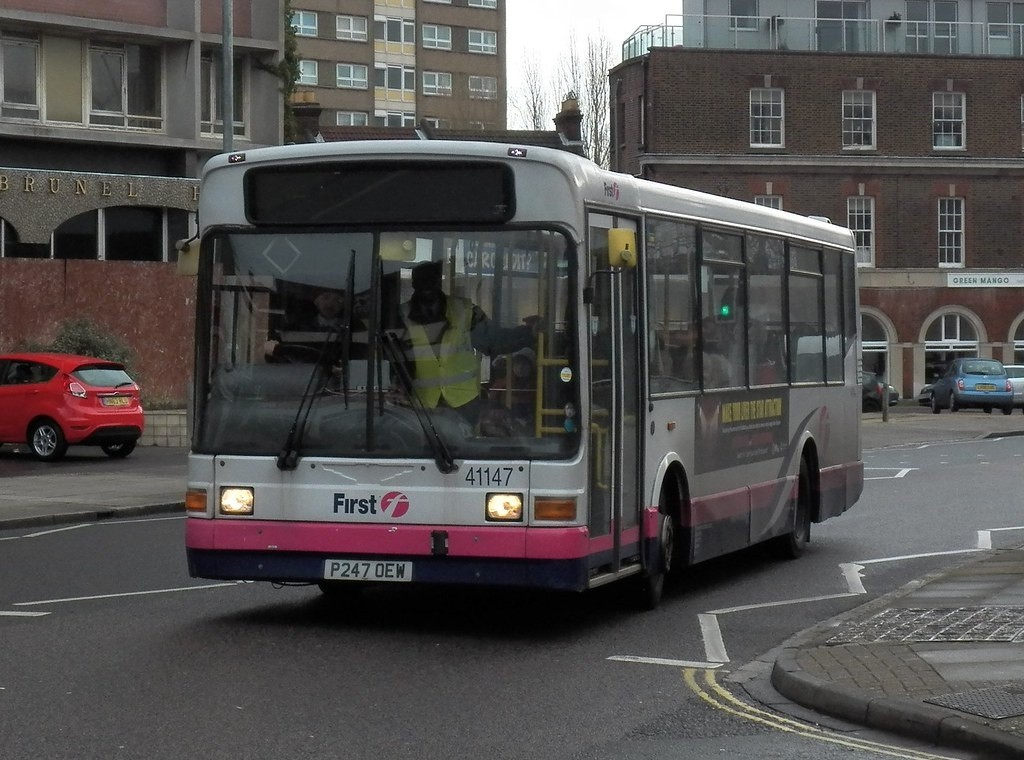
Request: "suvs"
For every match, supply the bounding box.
[993,364,1024,415]
[0,352,144,463]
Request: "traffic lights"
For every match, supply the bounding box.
[720,285,734,322]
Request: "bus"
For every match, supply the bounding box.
[175,138,865,610]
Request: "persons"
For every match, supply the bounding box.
[264,280,368,364]
[393,262,543,427]
[655,288,767,394]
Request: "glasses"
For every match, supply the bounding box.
[322,294,345,304]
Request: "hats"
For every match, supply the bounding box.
[310,288,346,301]
[512,347,536,370]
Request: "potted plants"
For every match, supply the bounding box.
[886,10,902,27]
[767,15,784,29]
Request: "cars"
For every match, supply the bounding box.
[861,371,899,411]
[929,358,1013,415]
